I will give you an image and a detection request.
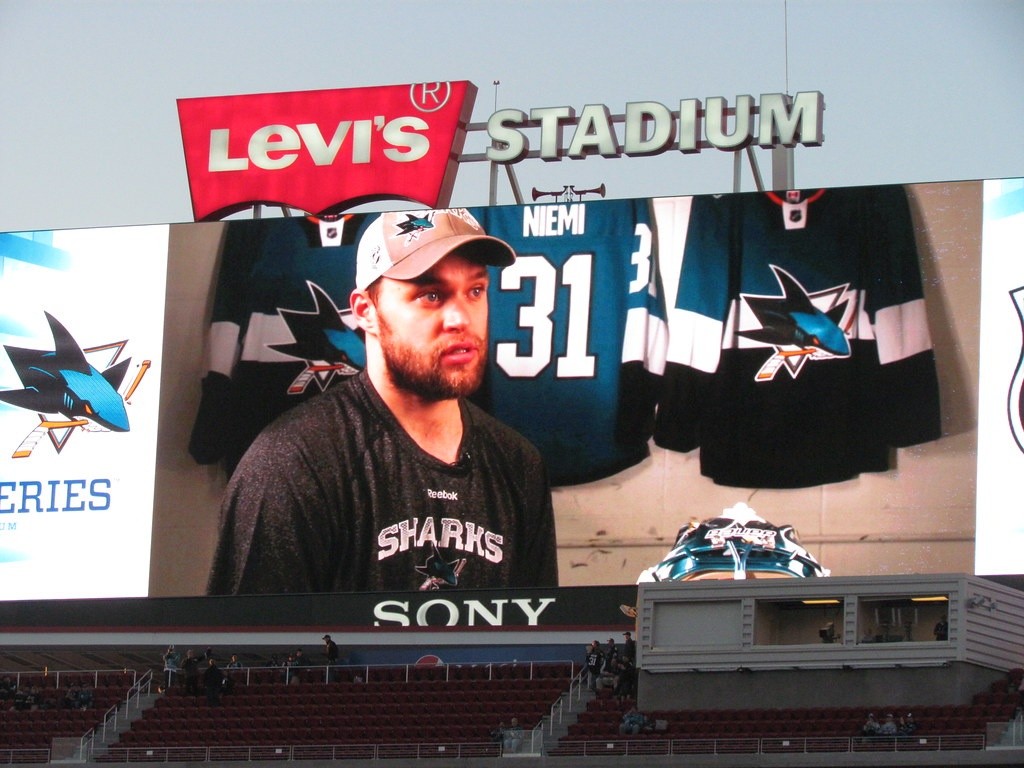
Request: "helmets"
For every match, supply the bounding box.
[635,517,822,584]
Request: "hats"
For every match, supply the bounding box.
[906,713,914,717]
[356,208,516,292]
[322,635,331,640]
[288,653,295,657]
[886,714,893,718]
[622,632,631,635]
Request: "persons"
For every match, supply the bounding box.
[209,209,559,595]
[933,614,949,640]
[490,717,521,755]
[0,674,99,711]
[163,644,244,707]
[861,711,918,745]
[618,704,645,736]
[586,632,636,710]
[267,628,339,683]
[820,622,843,643]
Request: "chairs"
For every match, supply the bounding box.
[0,666,1023,757]
